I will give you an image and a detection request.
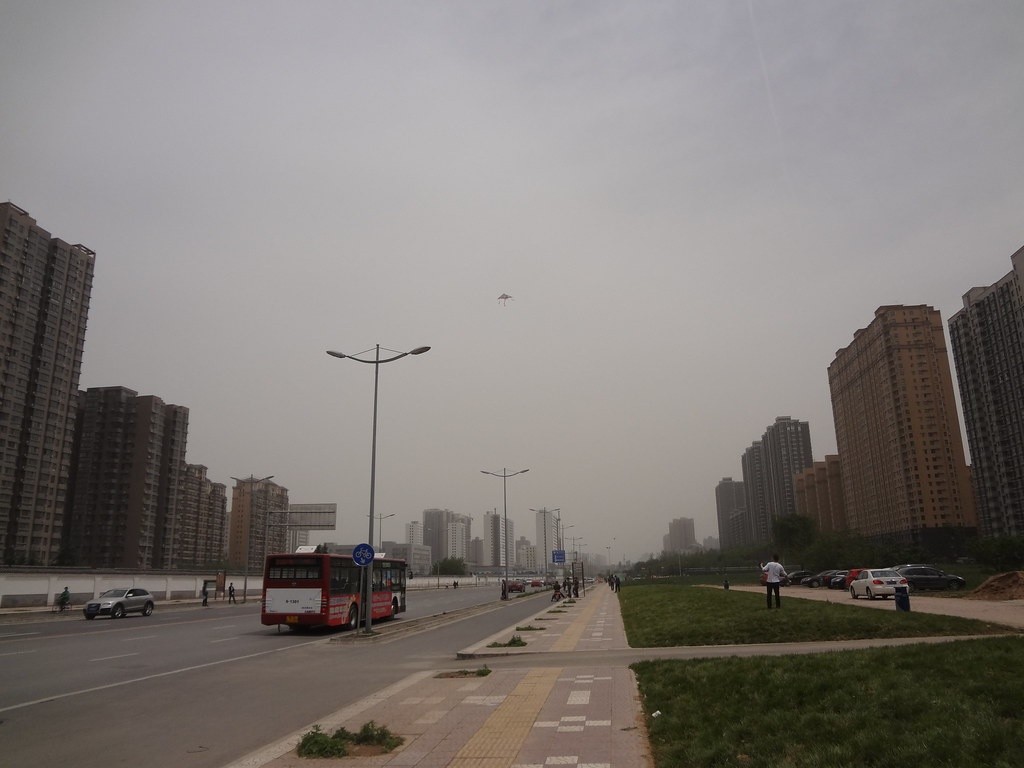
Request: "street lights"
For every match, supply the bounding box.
[326,343,432,635]
[530,508,560,590]
[482,467,531,602]
[367,513,399,554]
[231,475,276,602]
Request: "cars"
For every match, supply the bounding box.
[526,577,551,588]
[508,579,526,593]
[761,568,867,591]
[896,565,965,592]
[82,588,156,621]
[849,568,910,601]
[569,575,595,586]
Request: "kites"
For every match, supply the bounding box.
[497,293,512,307]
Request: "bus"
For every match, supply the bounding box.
[263,542,414,635]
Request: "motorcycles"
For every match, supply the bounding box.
[554,591,562,602]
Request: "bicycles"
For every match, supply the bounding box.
[52,596,73,615]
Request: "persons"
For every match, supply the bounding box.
[551,577,579,601]
[760,555,791,611]
[228,583,236,604]
[608,574,620,593]
[501,579,506,598]
[202,582,208,606]
[60,587,70,612]
